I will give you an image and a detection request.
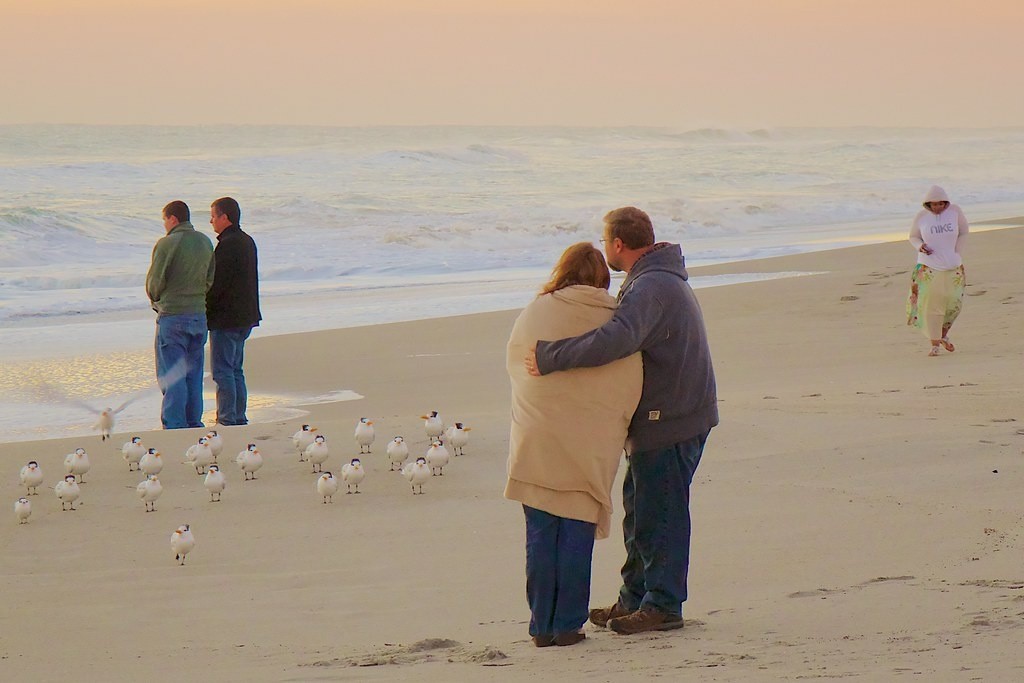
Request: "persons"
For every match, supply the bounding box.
[204,196,263,426]
[503,242,645,647]
[145,200,216,430]
[523,205,718,634]
[905,184,970,357]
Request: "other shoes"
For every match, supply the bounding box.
[940,340,955,351]
[928,347,940,356]
[555,629,586,645]
[532,636,553,646]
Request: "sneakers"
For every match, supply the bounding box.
[589,602,636,629]
[607,610,684,634]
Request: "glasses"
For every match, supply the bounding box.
[599,239,610,245]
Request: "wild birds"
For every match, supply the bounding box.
[15,361,472,566]
